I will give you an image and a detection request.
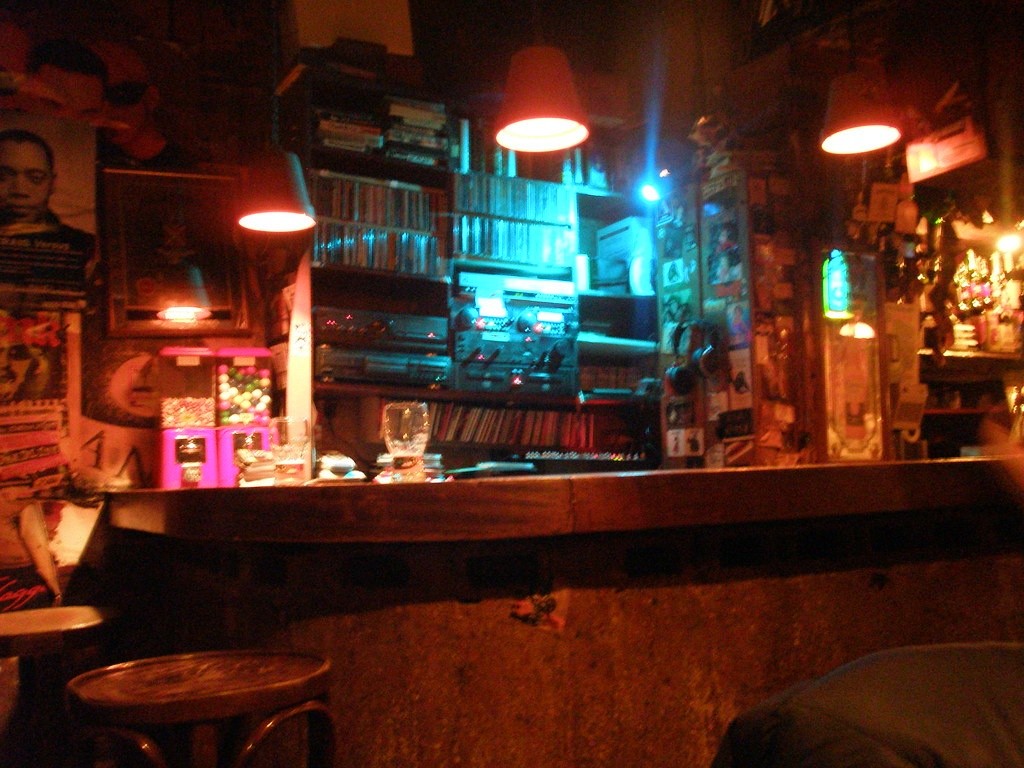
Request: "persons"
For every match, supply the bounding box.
[21,27,212,177]
[0,126,97,283]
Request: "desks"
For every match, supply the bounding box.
[102,454,1024,768]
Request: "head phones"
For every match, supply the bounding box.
[663,320,726,397]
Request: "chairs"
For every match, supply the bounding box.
[710,640,1024,768]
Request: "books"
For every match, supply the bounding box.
[312,90,641,192]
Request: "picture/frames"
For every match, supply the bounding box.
[103,164,257,342]
[0,107,102,315]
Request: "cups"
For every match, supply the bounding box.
[384,402,431,482]
[270,416,309,481]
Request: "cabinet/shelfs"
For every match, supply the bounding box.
[267,36,661,454]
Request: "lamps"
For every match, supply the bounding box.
[492,0,592,155]
[814,0,906,161]
[234,0,316,234]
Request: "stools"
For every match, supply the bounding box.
[0,605,129,661]
[61,645,337,768]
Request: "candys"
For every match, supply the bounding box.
[218,364,272,425]
